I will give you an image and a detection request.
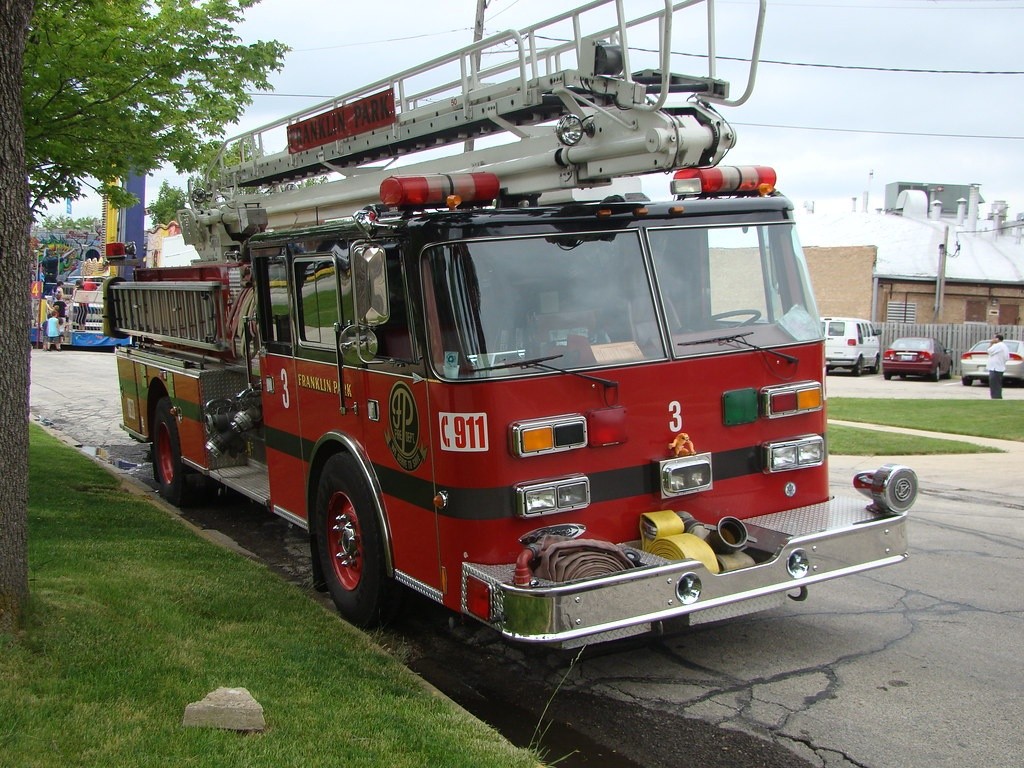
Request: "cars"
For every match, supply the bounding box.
[960,339,1024,387]
[881,337,953,382]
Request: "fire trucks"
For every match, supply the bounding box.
[98,0,918,670]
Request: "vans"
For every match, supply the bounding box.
[819,316,882,376]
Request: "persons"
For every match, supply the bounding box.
[52,281,64,303]
[53,292,68,317]
[72,278,103,330]
[42,303,65,351]
[986,335,1008,399]
